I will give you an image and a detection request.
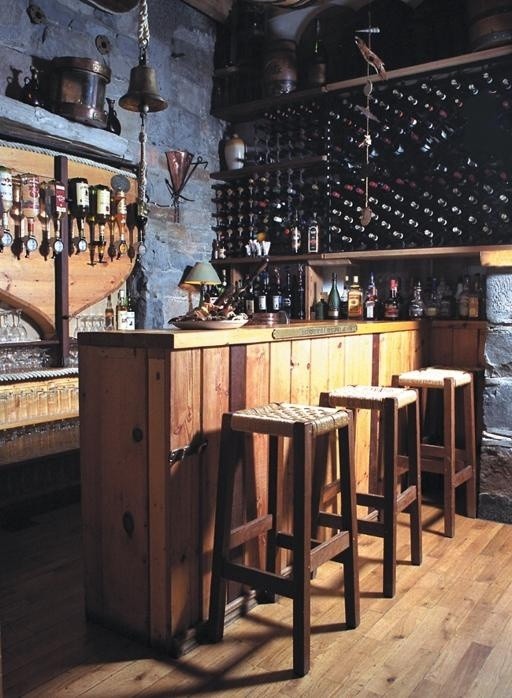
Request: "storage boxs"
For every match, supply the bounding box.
[77,319,489,662]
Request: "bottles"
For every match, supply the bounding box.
[134,196,148,244]
[95,183,111,243]
[68,177,91,240]
[427,279,441,320]
[272,215,293,227]
[264,101,322,119]
[347,275,363,320]
[0,166,13,233]
[340,275,349,319]
[459,274,470,321]
[362,272,381,320]
[314,298,327,320]
[286,187,307,201]
[271,272,283,313]
[47,182,67,242]
[327,272,342,320]
[325,72,512,254]
[441,289,457,321]
[218,234,229,259]
[288,208,303,255]
[294,271,305,320]
[244,280,257,318]
[283,272,293,319]
[236,155,281,167]
[114,188,127,242]
[305,209,321,255]
[20,173,40,239]
[383,278,403,322]
[272,201,289,211]
[469,273,485,321]
[257,272,269,312]
[258,200,271,210]
[208,283,221,307]
[409,280,426,321]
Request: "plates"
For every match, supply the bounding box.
[169,317,250,329]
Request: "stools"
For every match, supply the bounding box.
[204,400,361,677]
[391,366,479,540]
[305,383,423,600]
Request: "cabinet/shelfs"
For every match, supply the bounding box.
[209,42,510,260]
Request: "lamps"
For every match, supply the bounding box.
[184,261,222,307]
[177,266,199,313]
